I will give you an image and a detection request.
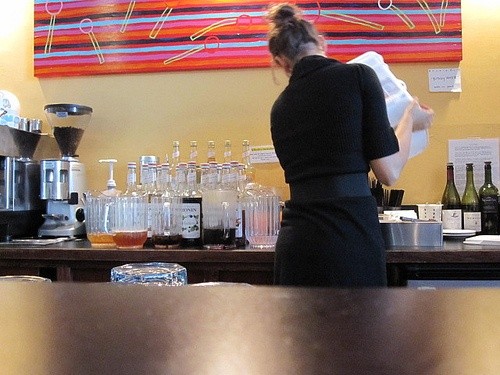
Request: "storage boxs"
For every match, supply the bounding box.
[0,125,62,161]
[380,220,445,245]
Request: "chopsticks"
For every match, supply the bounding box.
[369,179,383,206]
[384,188,404,206]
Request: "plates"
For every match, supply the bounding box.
[442,230,476,237]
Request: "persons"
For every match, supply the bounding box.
[268,3,435,287]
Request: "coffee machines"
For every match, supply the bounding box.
[37,103,93,239]
[0,113,40,241]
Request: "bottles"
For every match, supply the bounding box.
[207,141,218,163]
[139,156,159,185]
[171,140,181,165]
[240,140,257,183]
[122,161,256,246]
[461,163,482,235]
[478,161,500,235]
[441,162,461,229]
[222,140,233,164]
[188,141,198,162]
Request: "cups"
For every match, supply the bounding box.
[202,189,240,249]
[188,281,254,288]
[84,194,117,247]
[0,275,52,284]
[245,194,285,249]
[102,194,148,249]
[111,263,187,284]
